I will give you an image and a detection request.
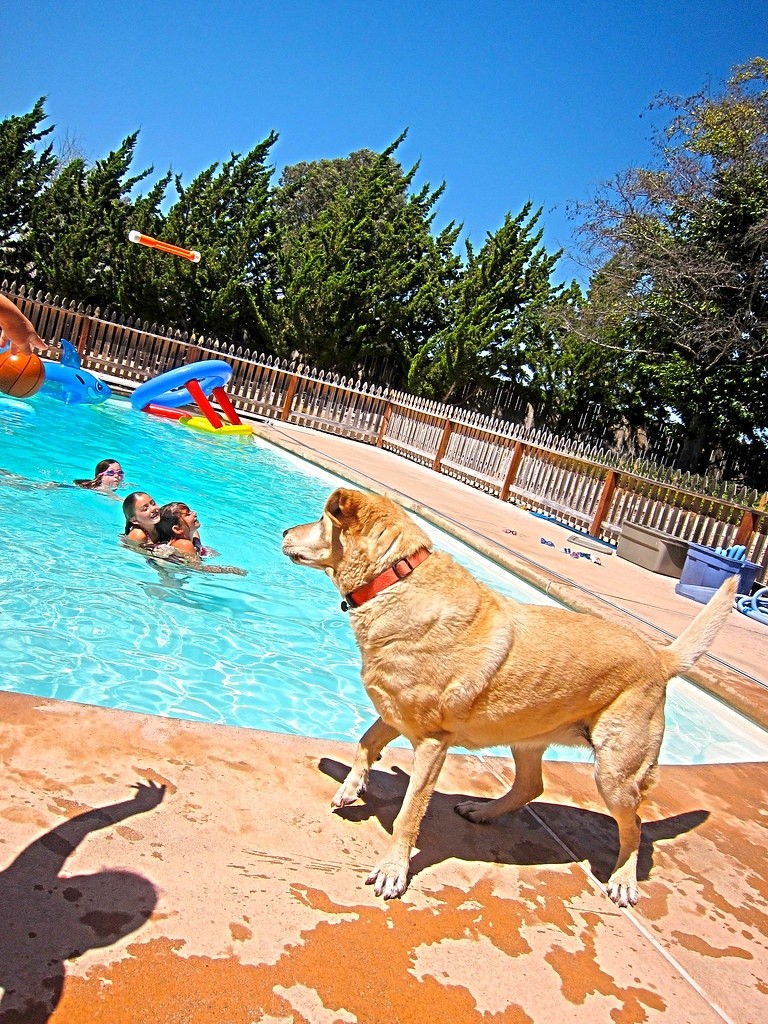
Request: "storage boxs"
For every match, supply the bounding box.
[675,541,764,605]
[616,520,689,579]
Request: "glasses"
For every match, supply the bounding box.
[505,529,517,535]
[98,469,124,477]
[564,547,602,565]
[541,538,553,546]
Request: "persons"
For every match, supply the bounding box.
[93,459,123,487]
[155,502,200,555]
[122,492,202,552]
[0,294,48,356]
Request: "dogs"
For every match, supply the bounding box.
[282,487,739,900]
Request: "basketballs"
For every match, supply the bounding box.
[0,349,47,398]
